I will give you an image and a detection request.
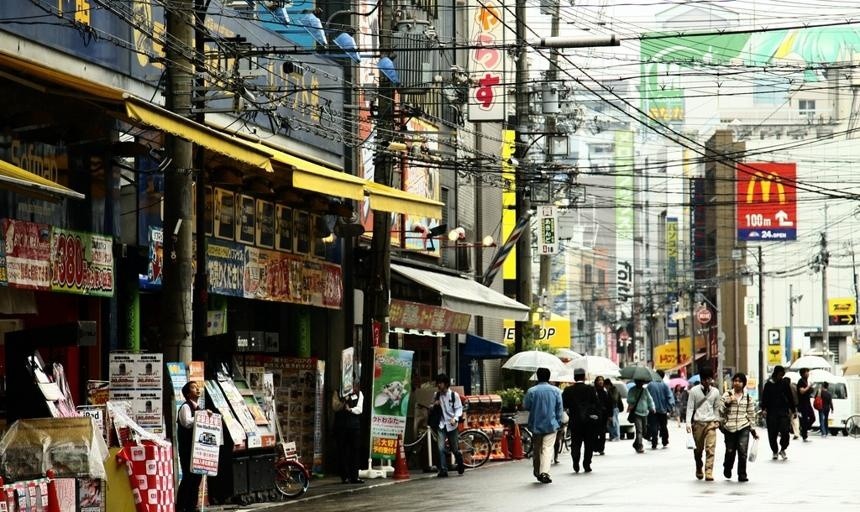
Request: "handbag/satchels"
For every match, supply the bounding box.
[814,396,823,410]
[428,405,442,430]
[628,408,637,423]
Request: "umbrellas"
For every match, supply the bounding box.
[783,355,832,386]
[839,352,860,377]
[500,347,662,387]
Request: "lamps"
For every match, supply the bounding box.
[365,225,497,251]
[150,0,399,85]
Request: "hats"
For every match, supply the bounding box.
[574,368,585,375]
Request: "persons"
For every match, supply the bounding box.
[627,369,675,453]
[175,380,214,512]
[523,368,620,484]
[330,370,366,484]
[383,380,405,400]
[80,478,101,508]
[275,370,315,432]
[427,373,467,479]
[761,366,834,460]
[673,368,754,483]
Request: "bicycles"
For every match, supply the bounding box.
[502,397,533,457]
[845,413,860,438]
[275,456,311,499]
[558,407,572,453]
[418,402,492,468]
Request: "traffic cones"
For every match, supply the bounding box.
[513,424,524,459]
[500,433,511,461]
[393,432,409,479]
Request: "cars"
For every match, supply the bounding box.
[604,380,634,440]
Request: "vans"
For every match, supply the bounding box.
[803,379,850,437]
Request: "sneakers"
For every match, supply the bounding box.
[351,478,364,484]
[772,451,778,458]
[738,477,748,481]
[724,467,731,478]
[438,472,449,478]
[697,468,704,479]
[458,464,464,474]
[779,451,787,459]
[633,441,644,453]
[705,473,713,480]
[535,473,552,483]
